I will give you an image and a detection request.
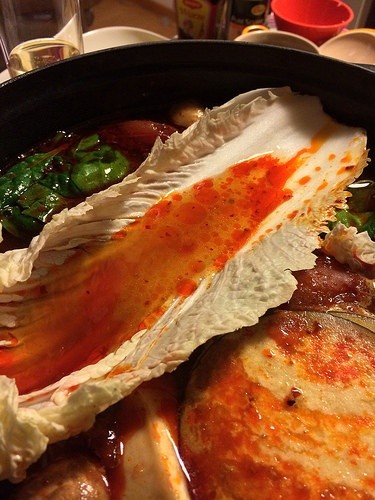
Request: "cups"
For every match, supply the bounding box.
[0,0,84,80]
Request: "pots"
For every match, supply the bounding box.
[0,41,375,187]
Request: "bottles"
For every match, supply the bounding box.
[174,0,231,39]
[228,0,268,40]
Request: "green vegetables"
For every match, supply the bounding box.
[1,129,131,242]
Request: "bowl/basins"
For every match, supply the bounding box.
[270,0,355,46]
[320,31,375,66]
[233,30,319,55]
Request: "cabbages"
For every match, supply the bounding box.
[0,88,370,484]
[320,221,375,272]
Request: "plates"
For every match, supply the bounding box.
[0,25,170,82]
